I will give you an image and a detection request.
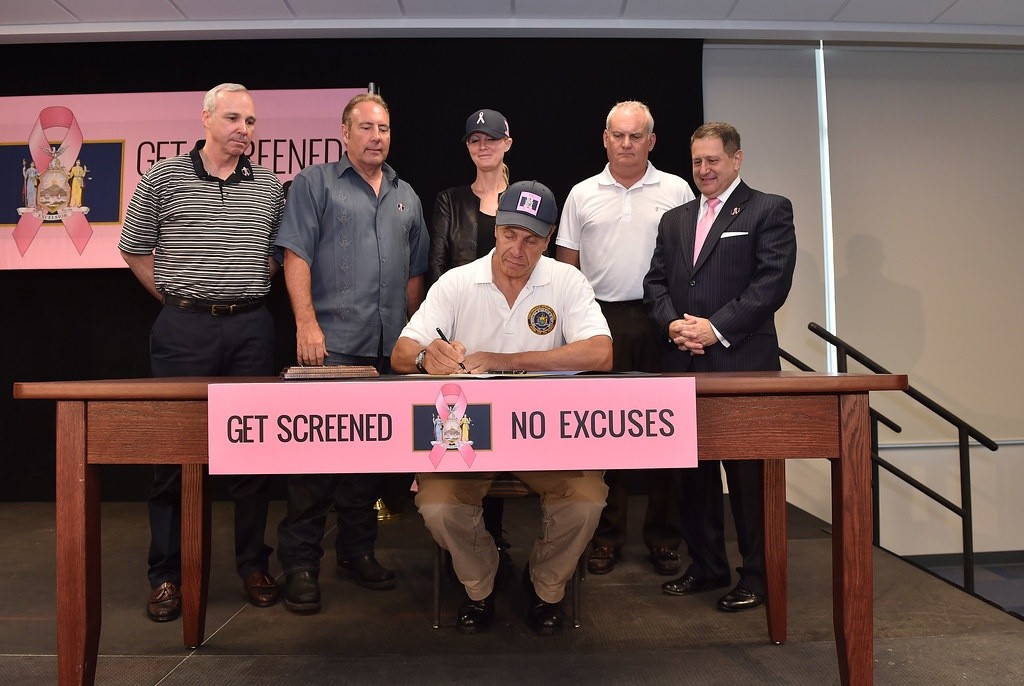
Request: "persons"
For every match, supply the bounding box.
[643,121,796,616]
[555,100,699,577]
[274,94,431,612]
[391,172,609,639]
[117,84,289,623]
[431,110,527,589]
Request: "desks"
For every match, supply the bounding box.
[14,371,910,686]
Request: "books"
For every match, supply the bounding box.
[280,365,379,380]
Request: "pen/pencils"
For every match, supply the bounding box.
[485,370,527,374]
[436,327,468,374]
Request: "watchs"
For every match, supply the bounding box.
[413,349,429,373]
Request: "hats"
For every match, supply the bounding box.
[462,109,510,140]
[496,181,558,238]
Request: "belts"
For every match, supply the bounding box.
[164,295,267,316]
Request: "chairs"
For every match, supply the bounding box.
[432,480,585,629]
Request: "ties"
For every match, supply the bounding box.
[693,198,723,268]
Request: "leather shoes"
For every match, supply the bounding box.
[280,570,322,613]
[520,560,565,636]
[716,581,765,612]
[588,544,624,575]
[243,567,281,608]
[456,592,494,634]
[661,565,732,595]
[335,550,397,589]
[146,580,182,623]
[649,547,682,575]
